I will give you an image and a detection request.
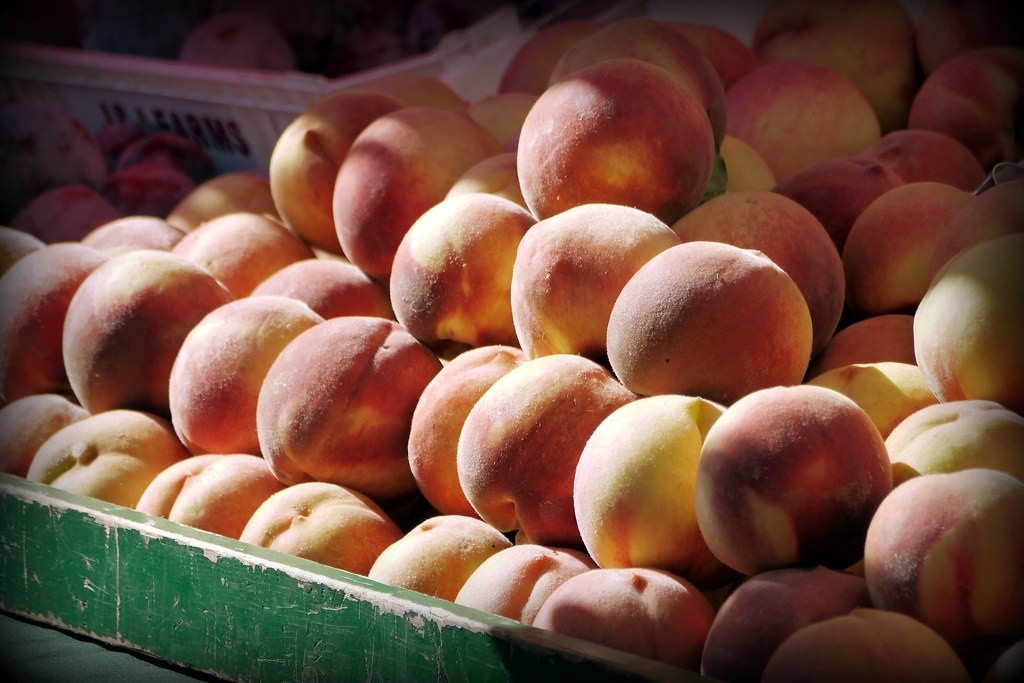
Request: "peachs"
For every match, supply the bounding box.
[0,0,1024,683]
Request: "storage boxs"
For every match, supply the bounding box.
[0,1,523,173]
